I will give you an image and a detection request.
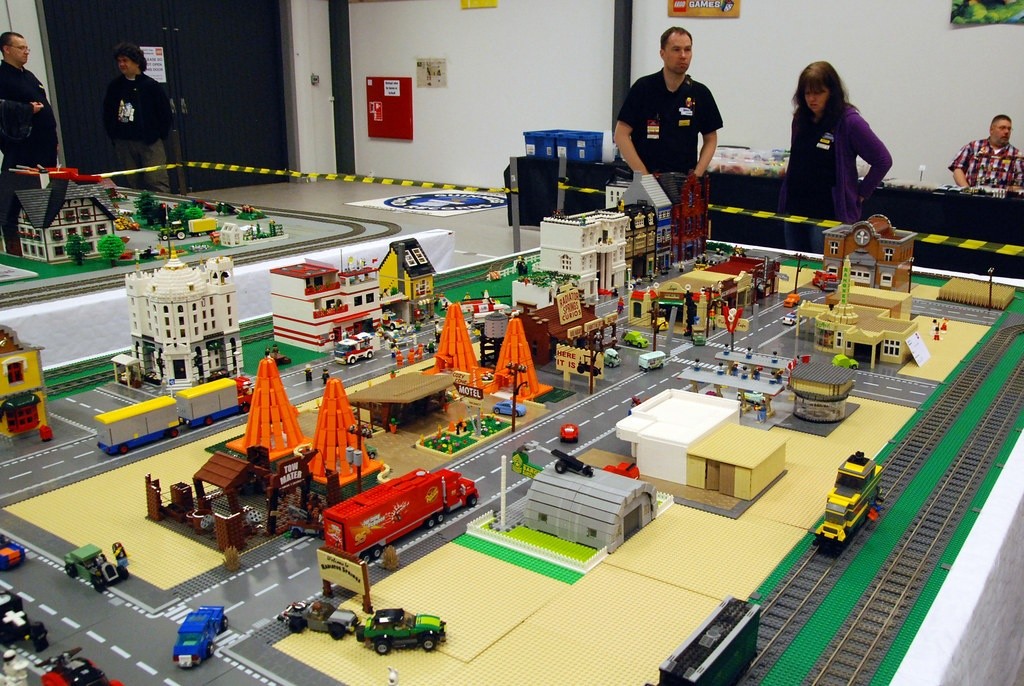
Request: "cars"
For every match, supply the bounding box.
[831,354,859,369]
[560,422,579,443]
[605,349,622,368]
[493,400,525,417]
[0,533,132,686]
[624,331,649,348]
[173,605,228,668]
[782,293,803,325]
[736,390,764,405]
[603,460,640,480]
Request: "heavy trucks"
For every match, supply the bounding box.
[322,467,482,564]
[93,376,259,455]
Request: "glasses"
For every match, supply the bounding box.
[7,45,30,51]
[995,126,1013,132]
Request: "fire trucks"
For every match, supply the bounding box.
[383,309,408,330]
[333,332,375,365]
[812,268,839,291]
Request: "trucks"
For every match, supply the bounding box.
[813,450,886,556]
[645,593,762,686]
[691,324,706,346]
[159,217,217,241]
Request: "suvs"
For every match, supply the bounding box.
[357,608,447,655]
[276,600,362,641]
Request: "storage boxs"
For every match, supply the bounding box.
[555,131,604,161]
[523,129,576,157]
[700,146,790,177]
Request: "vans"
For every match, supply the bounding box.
[637,351,666,372]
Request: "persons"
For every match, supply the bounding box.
[779,61,892,253]
[948,113,1023,197]
[103,44,172,194]
[0,31,60,173]
[613,29,723,180]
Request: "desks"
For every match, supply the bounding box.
[511,156,1024,281]
